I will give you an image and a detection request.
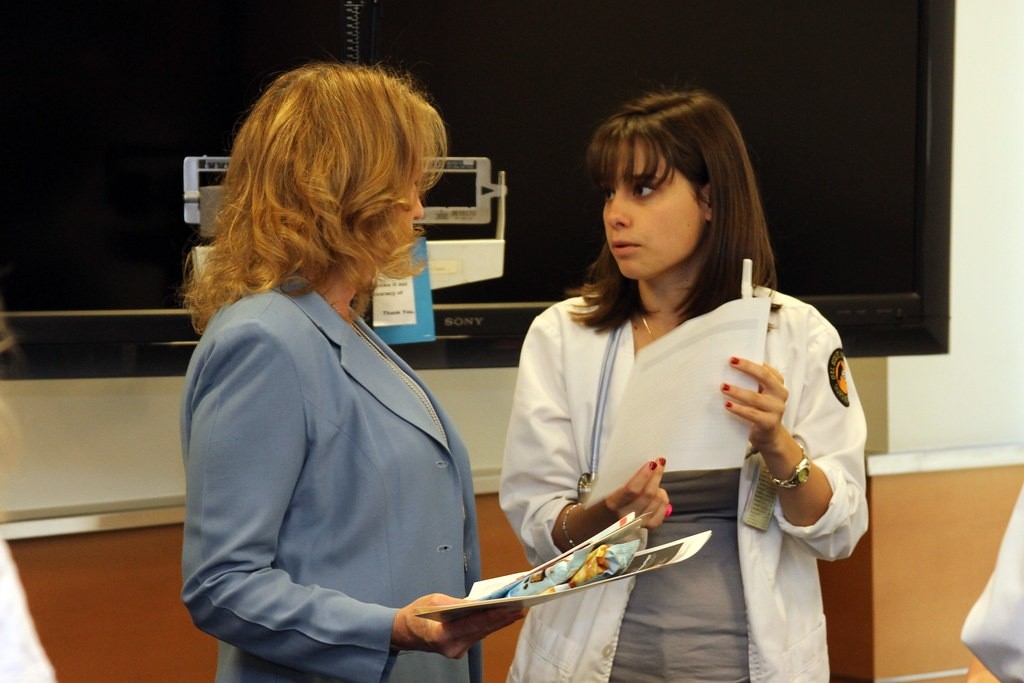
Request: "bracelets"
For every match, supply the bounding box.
[562,502,582,548]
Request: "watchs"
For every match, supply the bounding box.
[766,444,811,489]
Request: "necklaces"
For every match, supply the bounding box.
[641,315,655,341]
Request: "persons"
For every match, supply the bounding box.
[176,62,532,683]
[495,87,872,683]
